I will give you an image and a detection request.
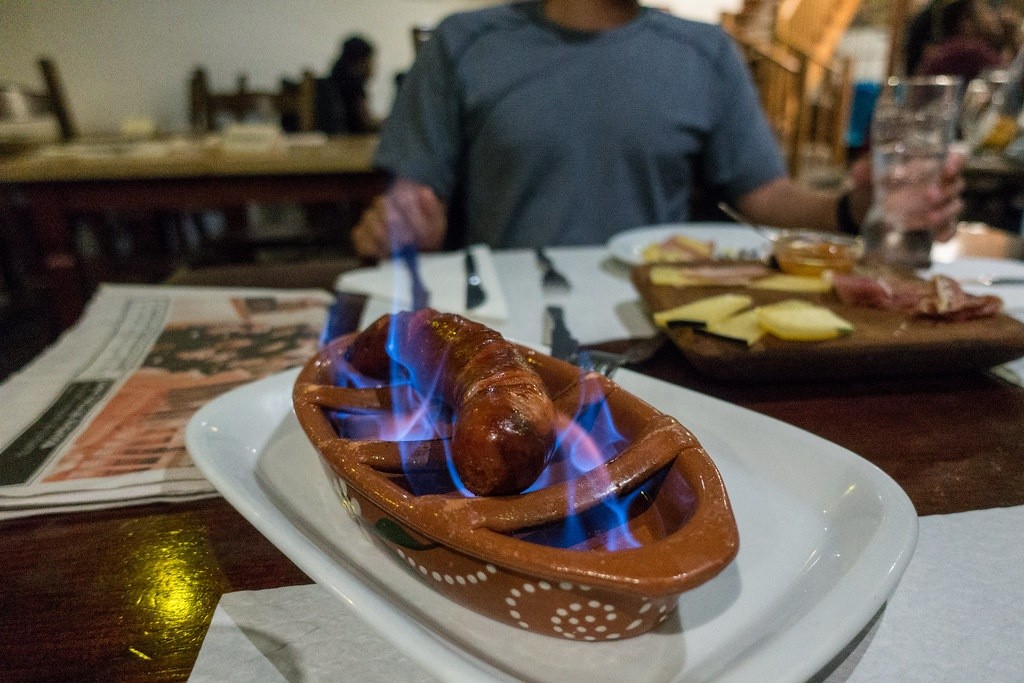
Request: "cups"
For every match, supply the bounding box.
[869,73,962,230]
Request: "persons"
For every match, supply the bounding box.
[353,1,966,269]
[904,0,1024,103]
[322,37,373,136]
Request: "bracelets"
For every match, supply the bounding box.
[838,195,860,235]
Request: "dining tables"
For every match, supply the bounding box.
[0,249,1024,683]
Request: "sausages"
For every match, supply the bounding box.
[343,308,556,495]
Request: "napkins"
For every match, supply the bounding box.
[332,243,510,322]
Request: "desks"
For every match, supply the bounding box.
[0,135,376,328]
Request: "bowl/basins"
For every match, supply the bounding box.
[774,228,869,276]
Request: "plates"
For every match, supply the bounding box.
[182,362,919,683]
[292,324,744,596]
[608,220,787,267]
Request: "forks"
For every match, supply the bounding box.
[567,333,668,380]
